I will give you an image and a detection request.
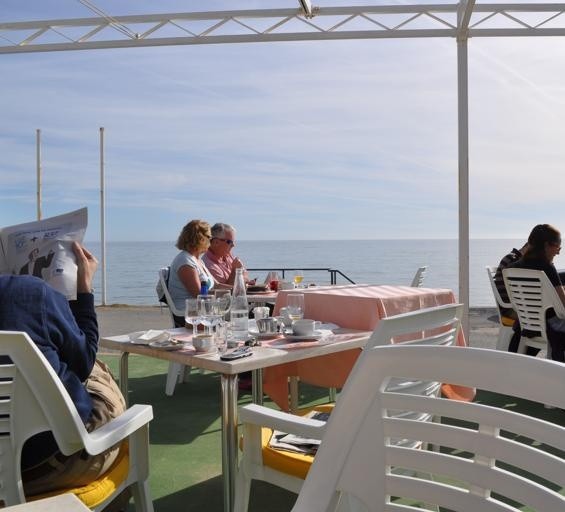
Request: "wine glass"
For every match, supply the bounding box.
[213,288,233,351]
[293,271,303,287]
[199,300,222,335]
[195,294,215,335]
[184,300,202,336]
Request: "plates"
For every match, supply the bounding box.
[148,339,184,350]
[246,291,270,295]
[250,331,279,338]
[283,330,321,339]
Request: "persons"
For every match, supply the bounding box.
[494,241,530,319]
[168,219,252,392]
[20,248,55,282]
[201,222,274,291]
[506,224,565,354]
[0,241,127,495]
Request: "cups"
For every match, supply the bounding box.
[192,335,214,353]
[286,293,306,320]
[292,320,323,336]
[269,272,279,291]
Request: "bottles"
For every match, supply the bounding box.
[200,281,209,295]
[280,307,291,326]
[253,306,270,321]
[275,316,284,331]
[229,267,249,342]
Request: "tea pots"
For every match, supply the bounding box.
[256,313,282,333]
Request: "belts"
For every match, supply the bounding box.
[22,424,94,484]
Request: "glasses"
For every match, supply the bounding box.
[204,234,212,240]
[215,236,234,245]
[550,243,562,252]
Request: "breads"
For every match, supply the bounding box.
[247,285,267,292]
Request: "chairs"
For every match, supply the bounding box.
[410,266,430,287]
[233,303,464,512]
[290,344,565,511]
[488,268,512,343]
[159,267,186,396]
[0,331,154,512]
[502,268,565,359]
